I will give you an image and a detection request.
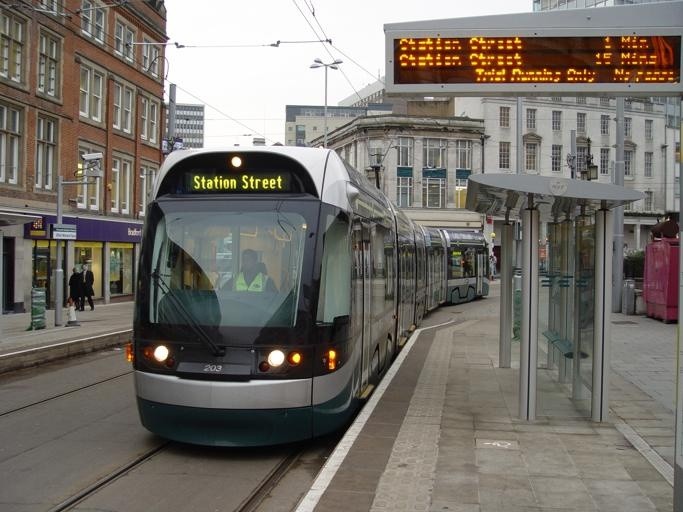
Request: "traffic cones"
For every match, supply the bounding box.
[64,298,81,327]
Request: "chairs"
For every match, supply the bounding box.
[242,263,267,274]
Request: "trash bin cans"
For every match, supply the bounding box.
[622,280,635,314]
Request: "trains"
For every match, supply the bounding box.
[124,146,490,449]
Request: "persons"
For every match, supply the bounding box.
[223,249,278,293]
[80,264,95,311]
[69,267,82,310]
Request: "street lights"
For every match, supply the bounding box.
[309,57,344,148]
[586,137,599,182]
